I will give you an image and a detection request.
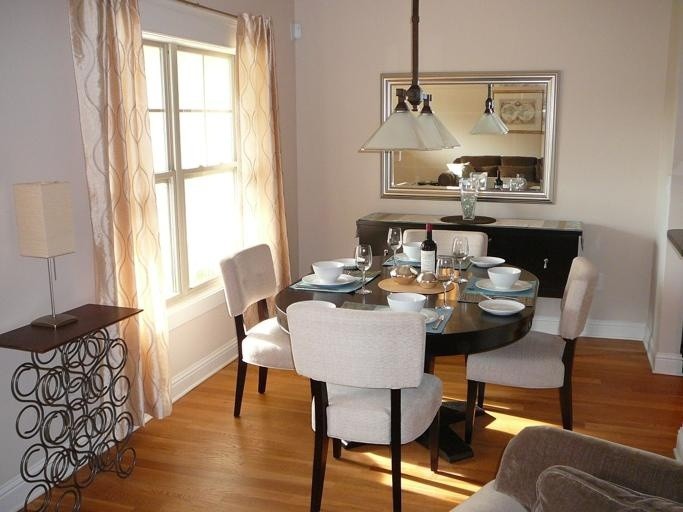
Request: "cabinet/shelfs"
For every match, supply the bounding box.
[355,213,584,301]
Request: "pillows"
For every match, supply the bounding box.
[446,161,477,184]
[531,464,682,512]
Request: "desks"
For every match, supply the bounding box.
[1,305,145,510]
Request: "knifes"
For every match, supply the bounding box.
[432,315,445,330]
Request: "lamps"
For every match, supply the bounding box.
[467,81,509,135]
[15,179,79,330]
[357,0,462,154]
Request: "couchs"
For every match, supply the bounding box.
[443,423,682,512]
[438,155,543,187]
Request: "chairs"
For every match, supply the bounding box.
[281,301,445,511]
[403,228,487,264]
[466,256,595,447]
[222,242,298,420]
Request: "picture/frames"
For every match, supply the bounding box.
[493,88,543,135]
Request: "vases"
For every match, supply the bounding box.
[469,172,488,190]
[459,178,479,219]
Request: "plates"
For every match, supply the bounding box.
[286,299,336,312]
[375,306,439,326]
[477,298,526,317]
[469,256,507,268]
[475,277,533,292]
[300,273,357,287]
[332,257,361,271]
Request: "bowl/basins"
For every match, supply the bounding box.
[310,260,344,283]
[487,265,522,289]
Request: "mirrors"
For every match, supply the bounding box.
[378,72,561,204]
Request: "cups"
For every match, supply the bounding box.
[461,163,527,191]
[458,177,478,221]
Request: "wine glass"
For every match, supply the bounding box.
[354,243,372,295]
[386,223,469,313]
